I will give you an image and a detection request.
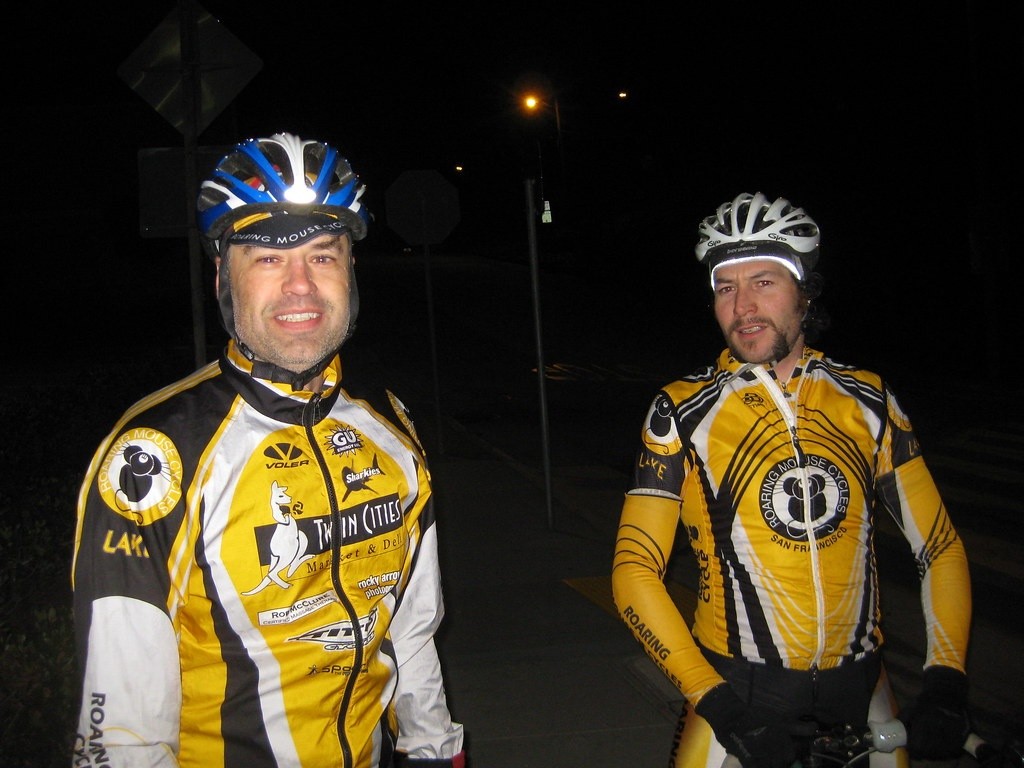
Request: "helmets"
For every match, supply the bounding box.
[196,133,369,241]
[694,192,821,282]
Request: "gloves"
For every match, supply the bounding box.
[895,665,970,763]
[692,683,818,768]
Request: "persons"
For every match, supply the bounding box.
[613,192,972,768]
[68,132,467,768]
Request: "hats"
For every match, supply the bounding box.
[219,169,354,249]
